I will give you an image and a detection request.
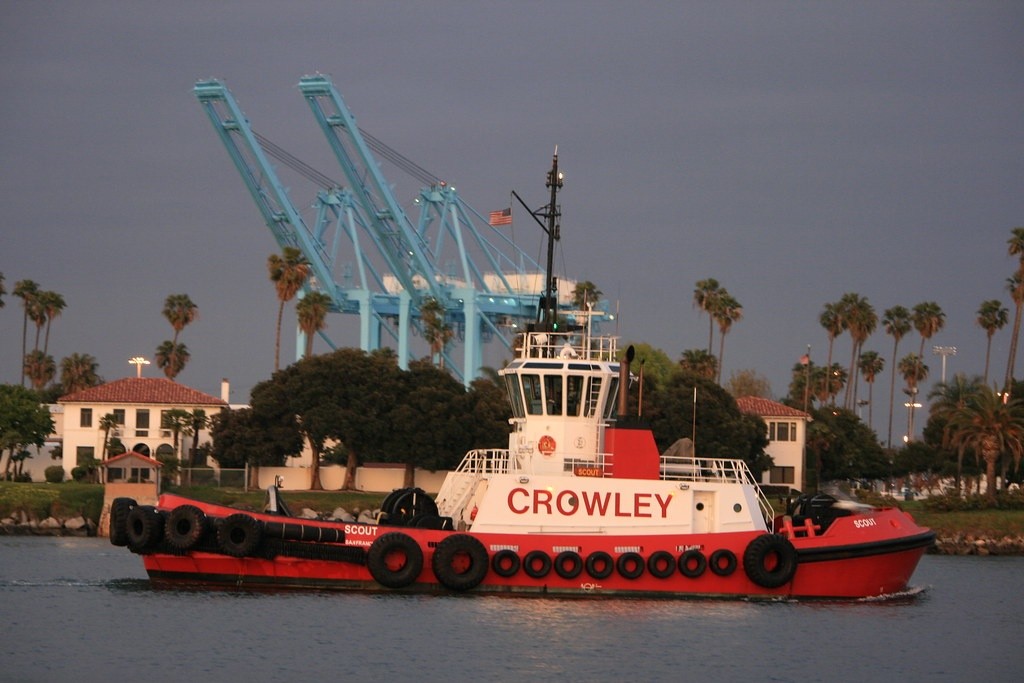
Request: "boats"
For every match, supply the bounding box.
[101,141,936,605]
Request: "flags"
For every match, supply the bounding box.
[489,208,512,225]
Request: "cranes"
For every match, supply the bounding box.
[192,69,612,398]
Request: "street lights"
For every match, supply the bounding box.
[932,345,957,394]
[904,403,922,444]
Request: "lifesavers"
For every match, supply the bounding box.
[492,550,520,577]
[165,503,208,550]
[126,506,164,548]
[648,550,677,579]
[367,532,423,589]
[678,550,707,577]
[616,552,644,579]
[216,513,262,557]
[585,550,613,579]
[432,534,489,590]
[522,550,551,578]
[743,534,799,588]
[555,551,582,578]
[709,548,738,576]
[108,497,137,547]
